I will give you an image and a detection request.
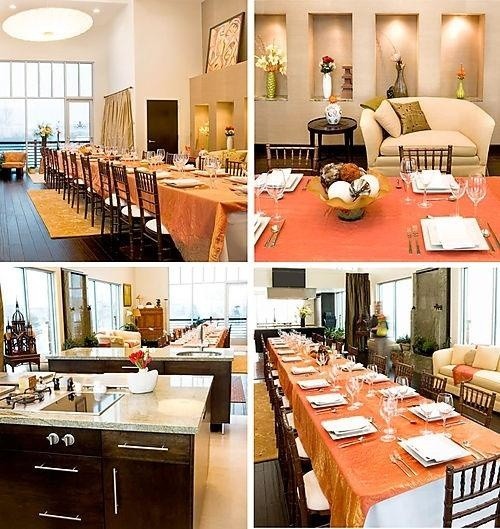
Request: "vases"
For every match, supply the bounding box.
[325,103,341,125]
[227,136,235,150]
[42,136,47,148]
[321,73,334,99]
[301,318,305,327]
[266,71,276,100]
[393,62,408,96]
[457,78,466,99]
[124,370,157,394]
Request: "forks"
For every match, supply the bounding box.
[406,223,420,254]
[301,179,311,190]
[388,448,417,478]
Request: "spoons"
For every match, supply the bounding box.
[427,196,457,202]
[462,438,486,459]
[263,224,278,249]
[482,228,496,251]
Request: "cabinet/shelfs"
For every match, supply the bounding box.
[135,308,163,343]
[1,424,104,527]
[100,432,193,528]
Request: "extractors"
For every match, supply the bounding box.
[265,267,317,301]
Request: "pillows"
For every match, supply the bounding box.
[372,100,401,137]
[391,102,432,134]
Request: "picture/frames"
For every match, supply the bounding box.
[123,283,132,307]
[205,12,245,76]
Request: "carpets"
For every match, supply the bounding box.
[28,172,82,186]
[255,381,284,464]
[232,353,247,373]
[231,375,247,404]
[27,188,137,240]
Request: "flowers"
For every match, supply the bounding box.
[376,30,404,66]
[254,32,287,77]
[225,125,236,137]
[318,56,336,74]
[329,96,337,103]
[296,300,312,318]
[34,124,55,137]
[454,62,470,79]
[128,349,153,371]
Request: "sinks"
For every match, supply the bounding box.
[175,350,223,357]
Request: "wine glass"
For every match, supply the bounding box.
[417,171,434,207]
[267,169,286,222]
[466,173,486,218]
[254,176,267,216]
[270,328,454,443]
[400,157,417,203]
[447,176,467,216]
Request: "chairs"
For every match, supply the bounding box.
[3,331,41,372]
[260,328,500,527]
[155,314,232,347]
[40,141,247,263]
[265,141,319,174]
[2,152,27,181]
[399,145,452,176]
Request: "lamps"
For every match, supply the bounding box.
[1,7,93,42]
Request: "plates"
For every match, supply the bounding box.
[254,173,304,192]
[58,142,247,192]
[408,405,461,423]
[170,332,220,345]
[411,168,458,193]
[397,439,473,468]
[421,218,490,252]
[254,217,271,246]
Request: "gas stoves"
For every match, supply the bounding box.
[0,386,59,413]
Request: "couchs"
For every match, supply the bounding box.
[97,330,142,348]
[361,98,496,173]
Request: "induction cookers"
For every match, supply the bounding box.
[45,389,122,452]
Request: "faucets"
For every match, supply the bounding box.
[200,323,210,351]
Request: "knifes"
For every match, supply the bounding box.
[270,219,287,246]
[339,436,378,448]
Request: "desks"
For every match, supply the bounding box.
[308,119,358,172]
[254,173,500,260]
